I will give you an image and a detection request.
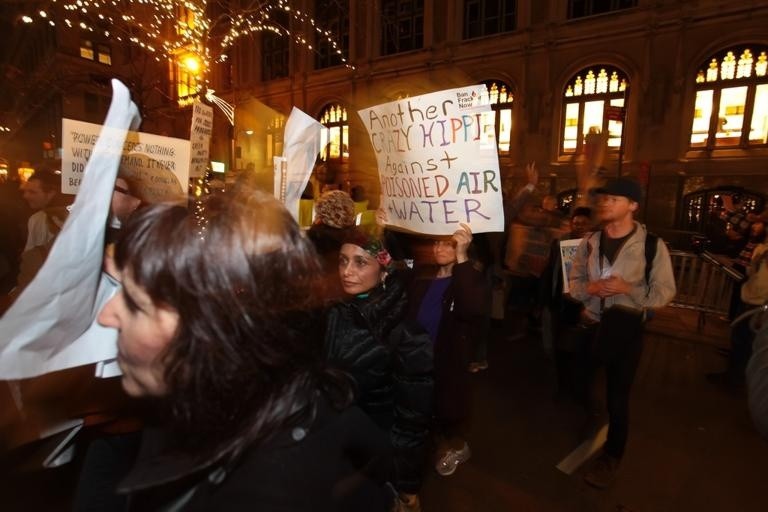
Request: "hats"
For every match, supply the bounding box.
[316,191,356,228]
[590,179,641,205]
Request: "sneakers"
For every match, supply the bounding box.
[436,441,470,476]
